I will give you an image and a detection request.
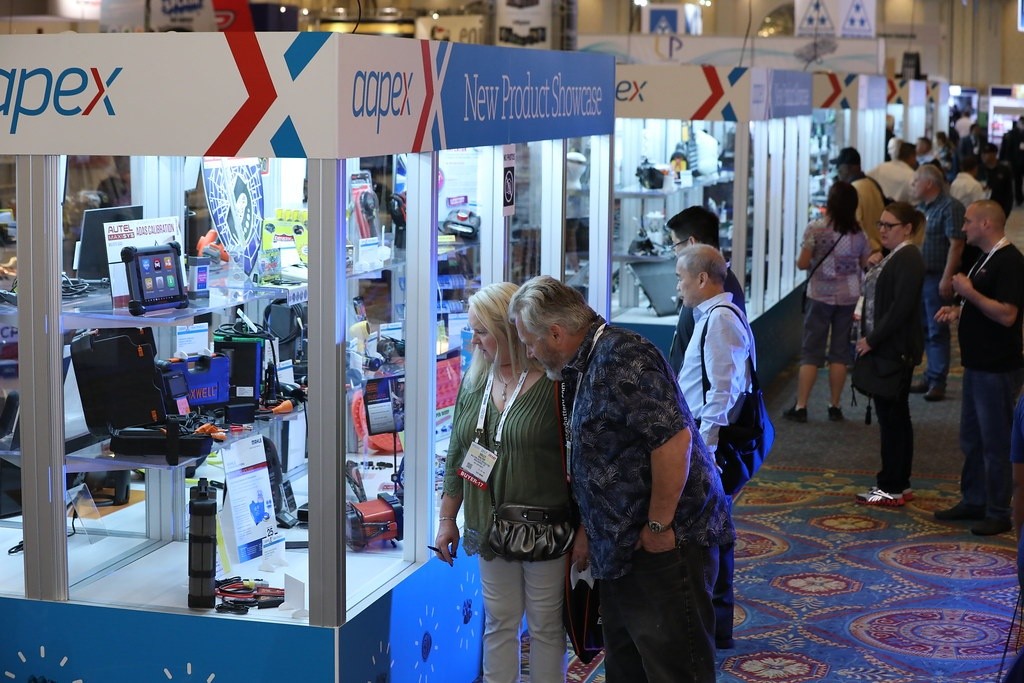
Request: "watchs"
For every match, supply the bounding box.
[647,519,672,534]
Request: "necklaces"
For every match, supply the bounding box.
[499,373,513,401]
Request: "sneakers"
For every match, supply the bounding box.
[856,490,904,507]
[828,406,844,420]
[870,486,913,500]
[783,405,807,423]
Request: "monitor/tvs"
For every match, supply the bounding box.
[76,205,145,282]
[121,242,188,316]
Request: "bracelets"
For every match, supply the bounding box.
[439,517,455,521]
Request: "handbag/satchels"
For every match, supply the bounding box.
[489,502,577,561]
[852,352,907,403]
[801,289,807,314]
[714,392,775,495]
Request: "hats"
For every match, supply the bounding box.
[981,143,998,153]
[829,148,860,165]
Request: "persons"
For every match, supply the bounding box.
[508,276,737,683]
[933,200,1024,536]
[676,243,756,648]
[866,122,1024,219]
[782,181,869,426]
[910,164,967,403]
[433,281,591,683]
[829,146,886,249]
[954,109,974,138]
[1010,394,1024,596]
[952,104,961,121]
[852,201,926,505]
[667,206,746,376]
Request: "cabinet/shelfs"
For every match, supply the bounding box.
[887,80,926,144]
[1,32,615,683]
[611,65,813,391]
[813,74,886,219]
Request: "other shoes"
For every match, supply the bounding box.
[935,502,986,519]
[972,518,1012,535]
[909,380,929,393]
[715,635,735,649]
[924,386,945,401]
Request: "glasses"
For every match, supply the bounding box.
[876,222,905,231]
[669,235,700,256]
